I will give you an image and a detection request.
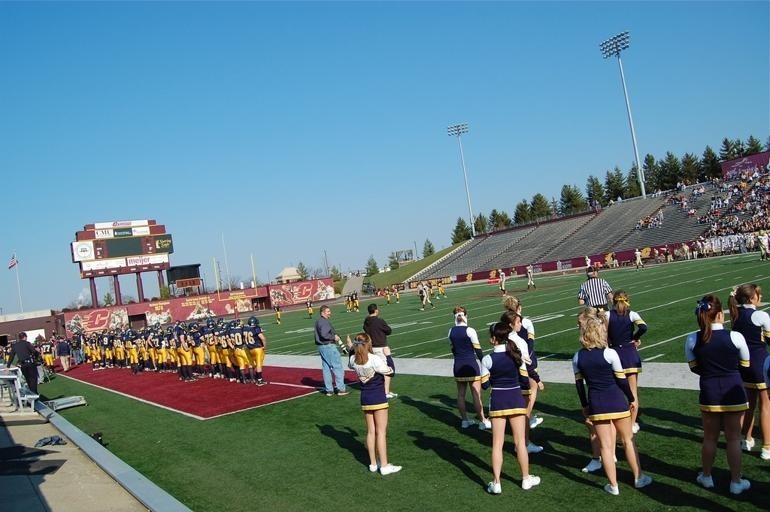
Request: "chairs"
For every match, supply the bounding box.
[0,364,40,415]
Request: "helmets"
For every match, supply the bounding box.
[166,316,257,331]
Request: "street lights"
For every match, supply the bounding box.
[446,120,476,237]
[598,30,650,199]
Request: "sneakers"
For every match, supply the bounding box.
[635,474,652,488]
[488,481,501,494]
[632,423,639,433]
[326,392,335,396]
[479,418,491,430]
[338,391,350,396]
[461,419,475,428]
[730,479,750,494]
[697,472,714,487]
[386,392,397,398]
[742,437,755,450]
[369,463,402,475]
[527,442,544,453]
[582,460,602,473]
[522,475,540,490]
[760,448,769,460]
[529,417,543,428]
[604,483,619,494]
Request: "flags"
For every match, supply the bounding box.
[8,254,17,269]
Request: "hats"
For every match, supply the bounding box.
[586,265,599,272]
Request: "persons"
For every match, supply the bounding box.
[0,317,267,394]
[612,252,619,268]
[393,286,400,303]
[313,305,350,395]
[497,269,507,295]
[500,312,544,454]
[274,301,281,325]
[385,287,391,304]
[344,291,359,313]
[685,293,751,494]
[305,298,314,319]
[577,268,614,312]
[482,322,541,494]
[618,196,622,203]
[347,332,402,475]
[449,305,491,430]
[504,297,544,430]
[416,279,448,311]
[585,255,591,268]
[364,303,398,398]
[573,290,654,496]
[634,165,769,272]
[609,199,615,207]
[728,283,770,460]
[527,264,536,289]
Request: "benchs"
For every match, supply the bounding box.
[670,178,754,243]
[410,225,535,282]
[535,194,670,264]
